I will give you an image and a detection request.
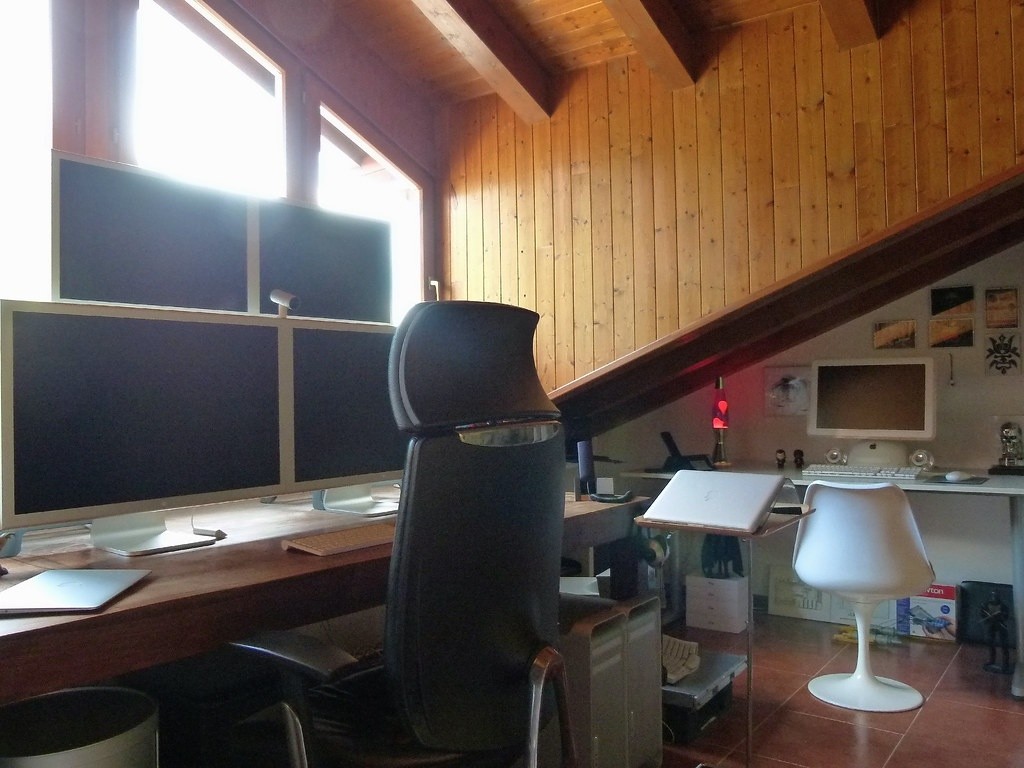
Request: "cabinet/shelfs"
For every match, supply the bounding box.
[685,572,748,634]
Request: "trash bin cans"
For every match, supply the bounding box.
[2,686,160,767]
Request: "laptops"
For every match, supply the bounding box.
[0,569,152,614]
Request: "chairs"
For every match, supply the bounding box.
[223,302,580,768]
[790,479,934,713]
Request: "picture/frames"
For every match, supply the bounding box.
[830,592,897,628]
[767,566,830,622]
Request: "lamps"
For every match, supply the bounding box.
[712,376,731,466]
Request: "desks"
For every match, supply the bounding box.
[0,490,652,768]
[619,467,1023,702]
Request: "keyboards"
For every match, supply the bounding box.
[281,524,395,557]
[802,463,922,480]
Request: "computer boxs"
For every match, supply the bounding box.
[516,575,663,768]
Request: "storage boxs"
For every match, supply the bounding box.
[897,585,961,641]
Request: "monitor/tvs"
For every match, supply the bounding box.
[1,148,413,556]
[807,357,936,465]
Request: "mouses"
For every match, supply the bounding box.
[945,471,971,481]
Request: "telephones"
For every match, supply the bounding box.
[645,431,718,473]
[573,437,614,502]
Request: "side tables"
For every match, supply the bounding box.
[633,509,815,768]
